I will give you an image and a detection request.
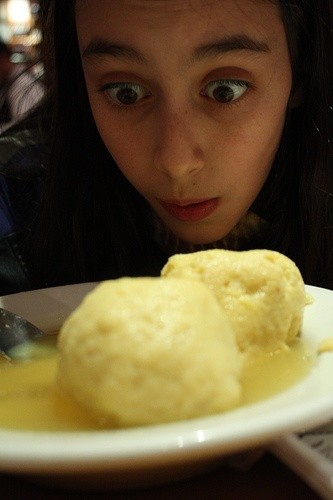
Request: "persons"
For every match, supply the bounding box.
[0,0,333,296]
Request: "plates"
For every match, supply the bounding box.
[0,279,333,474]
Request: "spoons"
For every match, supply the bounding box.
[0,308,55,361]
